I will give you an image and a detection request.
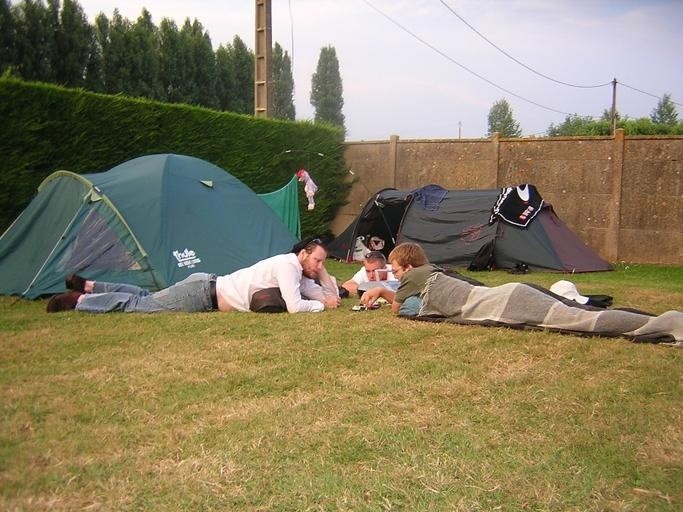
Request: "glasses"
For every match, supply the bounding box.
[312,238,323,244]
[365,253,384,259]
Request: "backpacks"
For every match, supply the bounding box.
[467,242,496,272]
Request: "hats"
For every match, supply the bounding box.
[549,279,589,305]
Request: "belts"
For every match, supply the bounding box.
[210,281,218,309]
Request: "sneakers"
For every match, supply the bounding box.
[65,273,86,292]
[46,291,81,312]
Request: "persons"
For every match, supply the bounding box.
[342,252,399,296]
[46,236,341,314]
[360,243,444,314]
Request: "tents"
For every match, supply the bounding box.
[325,184,615,273]
[0,154,301,309]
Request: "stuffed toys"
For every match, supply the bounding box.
[297,170,318,210]
